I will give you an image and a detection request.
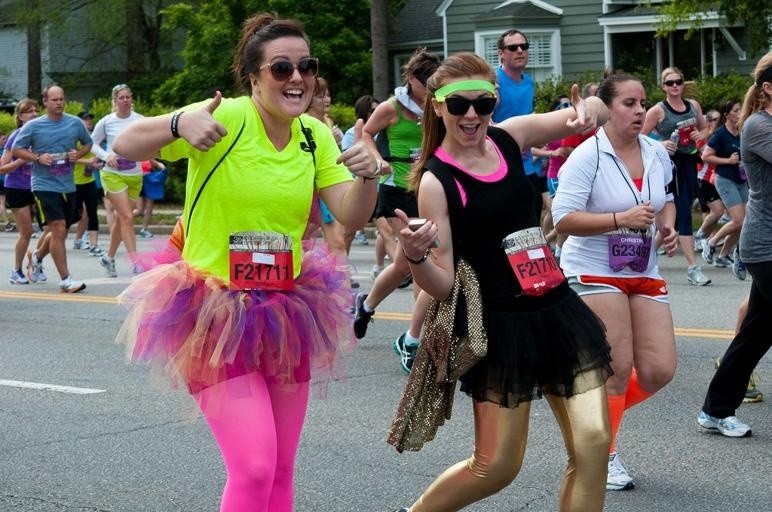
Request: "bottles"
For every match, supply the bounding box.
[666,129,683,155]
[737,160,746,181]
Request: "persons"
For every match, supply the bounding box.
[1,84,169,295]
[394,50,614,512]
[493,33,748,286]
[301,47,442,374]
[546,72,678,492]
[697,51,772,437]
[113,11,378,510]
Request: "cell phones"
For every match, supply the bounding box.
[408,219,440,248]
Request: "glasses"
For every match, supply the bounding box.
[260,58,321,81]
[505,43,529,51]
[663,78,683,86]
[445,98,497,116]
[556,103,572,109]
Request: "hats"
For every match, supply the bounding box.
[77,112,94,119]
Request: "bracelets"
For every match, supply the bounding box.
[401,250,427,264]
[362,159,382,184]
[170,110,183,138]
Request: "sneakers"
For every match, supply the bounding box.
[604,454,634,492]
[350,232,420,373]
[4,222,144,292]
[658,230,746,285]
[716,356,763,402]
[698,413,752,438]
